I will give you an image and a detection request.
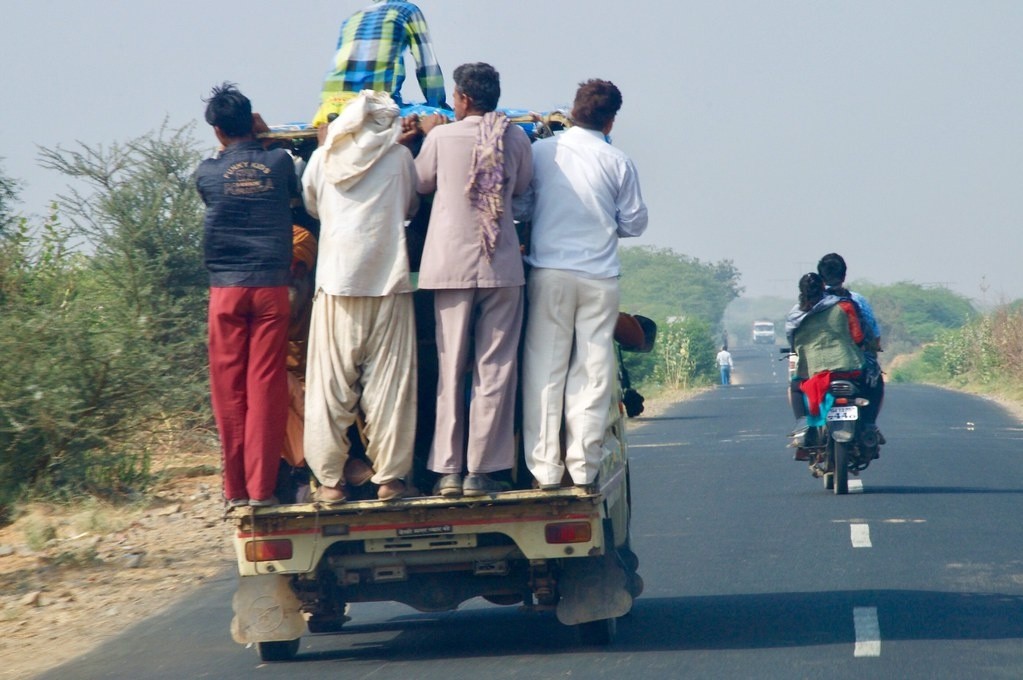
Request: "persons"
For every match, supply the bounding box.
[280,224,374,486]
[301,88,415,503]
[414,62,533,496]
[196,80,302,507]
[786,287,881,438]
[722,330,728,346]
[716,346,733,385]
[784,253,886,461]
[512,79,649,490]
[313,0,453,129]
[785,272,864,438]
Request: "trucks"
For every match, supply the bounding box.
[220,113,658,661]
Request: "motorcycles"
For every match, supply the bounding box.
[778,347,885,494]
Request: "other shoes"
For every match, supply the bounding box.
[531,478,561,490]
[786,435,805,448]
[229,496,248,507]
[573,482,595,487]
[432,473,462,496]
[463,475,505,495]
[791,416,810,435]
[247,495,280,506]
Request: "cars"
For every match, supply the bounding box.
[753,323,775,344]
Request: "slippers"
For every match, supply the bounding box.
[378,477,420,501]
[317,485,347,503]
